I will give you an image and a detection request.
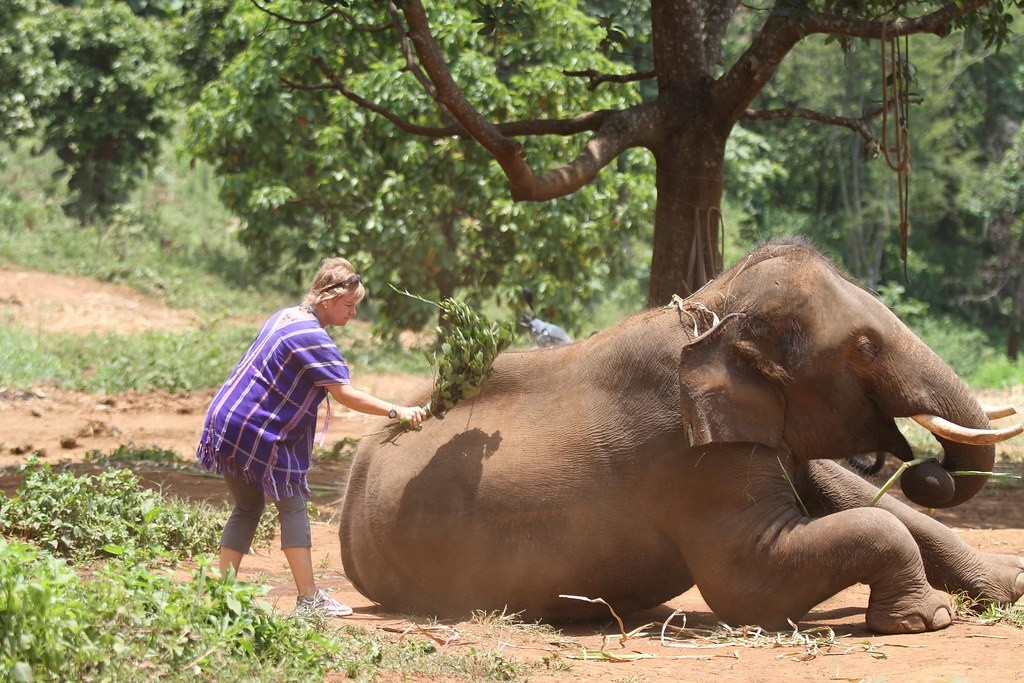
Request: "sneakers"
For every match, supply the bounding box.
[296,589,353,616]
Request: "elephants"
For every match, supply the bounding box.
[338,233,1024,637]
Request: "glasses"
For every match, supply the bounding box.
[320,275,361,294]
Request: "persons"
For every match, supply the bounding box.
[196,257,426,617]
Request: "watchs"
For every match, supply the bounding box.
[389,405,397,418]
